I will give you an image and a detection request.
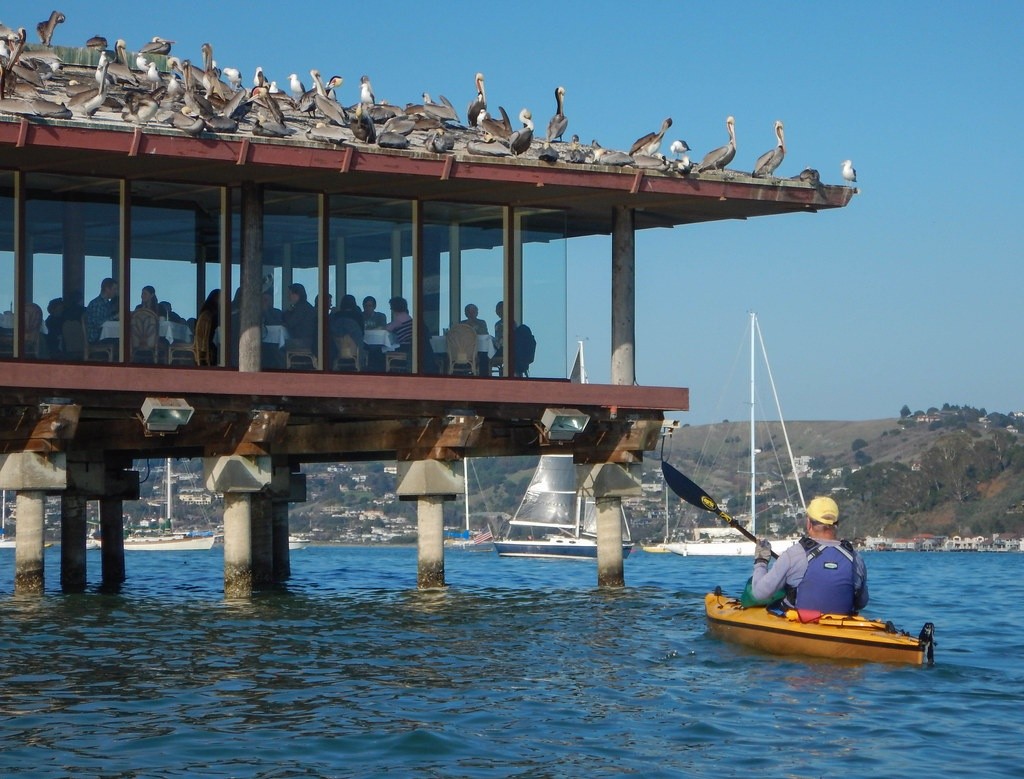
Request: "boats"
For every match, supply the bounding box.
[704,585,935,664]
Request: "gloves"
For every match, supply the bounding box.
[755,539,771,565]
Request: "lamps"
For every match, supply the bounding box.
[141,397,195,431]
[542,407,591,440]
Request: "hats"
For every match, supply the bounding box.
[807,497,839,524]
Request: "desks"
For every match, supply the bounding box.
[364,328,400,354]
[212,325,290,348]
[98,319,191,345]
[430,334,498,358]
[0,313,49,334]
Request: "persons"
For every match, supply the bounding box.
[87,277,120,345]
[385,298,412,352]
[361,296,386,329]
[461,304,488,336]
[233,286,283,324]
[45,298,65,336]
[135,286,159,316]
[752,496,869,615]
[339,295,361,312]
[160,301,183,322]
[490,301,518,358]
[21,303,43,346]
[281,284,333,363]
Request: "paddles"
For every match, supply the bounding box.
[660,460,780,560]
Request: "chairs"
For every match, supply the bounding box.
[0,307,537,378]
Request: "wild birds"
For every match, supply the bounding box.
[287,69,567,161]
[564,116,857,189]
[0,11,298,137]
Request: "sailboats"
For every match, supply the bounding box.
[638,317,809,557]
[0,490,17,547]
[445,459,495,552]
[489,341,634,559]
[86,460,217,552]
